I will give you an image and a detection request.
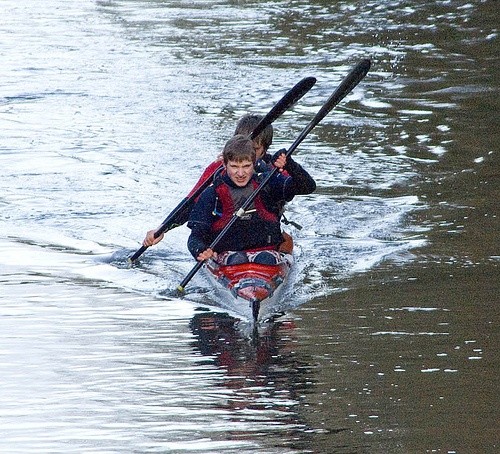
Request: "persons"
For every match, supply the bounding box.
[141,114,318,248]
[186,134,317,267]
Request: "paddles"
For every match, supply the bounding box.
[127,76,319,259]
[179,58,371,292]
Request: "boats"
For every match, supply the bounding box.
[200,229,293,322]
